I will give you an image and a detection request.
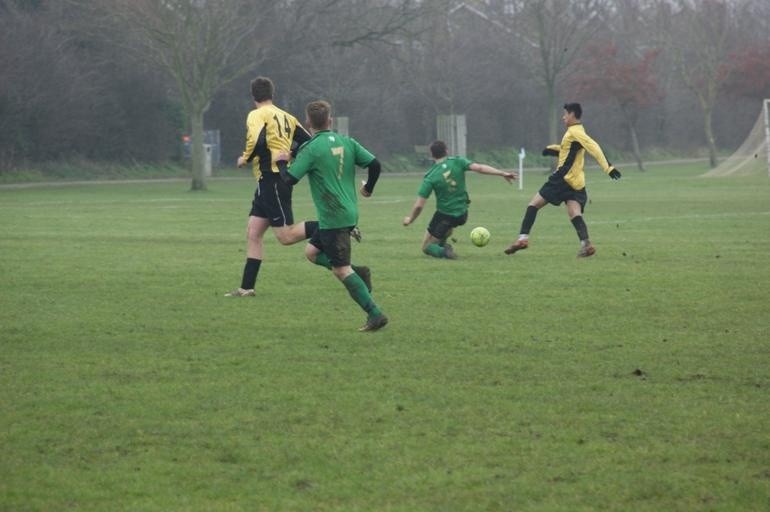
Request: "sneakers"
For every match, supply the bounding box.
[577,247,595,257]
[223,287,256,297]
[443,246,456,259]
[505,240,529,255]
[350,226,361,243]
[358,315,388,332]
[354,266,372,293]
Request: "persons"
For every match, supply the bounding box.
[275,100,389,332]
[404,140,520,260]
[224,77,361,296]
[504,103,622,259]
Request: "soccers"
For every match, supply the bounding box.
[471,227,490,247]
